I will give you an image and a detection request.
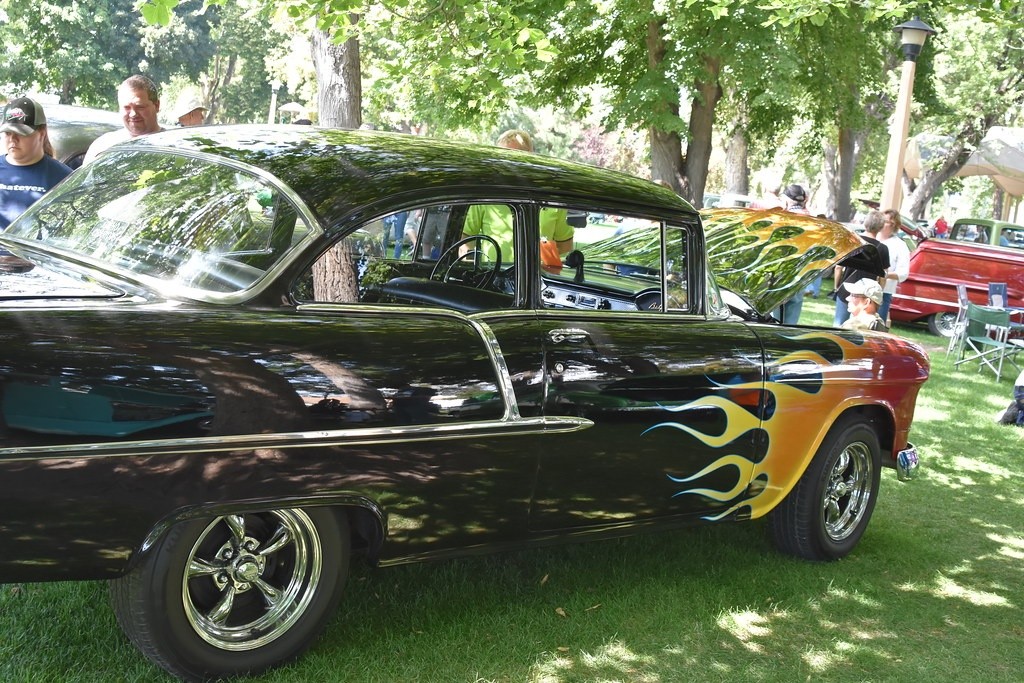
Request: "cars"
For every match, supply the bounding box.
[0,124,929,677]
[698,199,1023,343]
[0,102,259,261]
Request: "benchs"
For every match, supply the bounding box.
[113,240,266,289]
[383,277,515,314]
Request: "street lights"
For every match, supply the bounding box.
[878,15,936,244]
[268,75,282,124]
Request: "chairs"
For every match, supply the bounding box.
[986,283,1024,364]
[953,300,1021,382]
[946,285,1007,372]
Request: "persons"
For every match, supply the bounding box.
[773,185,809,324]
[383,212,407,260]
[805,214,829,298]
[174,95,209,126]
[974,225,988,243]
[0,95,73,233]
[933,216,947,238]
[404,208,424,256]
[1001,228,1010,246]
[878,209,910,323]
[1014,368,1024,428]
[82,75,167,169]
[840,279,885,331]
[421,206,451,258]
[458,132,573,265]
[833,213,891,328]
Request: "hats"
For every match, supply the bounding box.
[783,184,806,202]
[843,278,883,306]
[177,96,210,118]
[0,96,46,136]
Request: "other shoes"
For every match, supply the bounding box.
[995,402,1019,426]
